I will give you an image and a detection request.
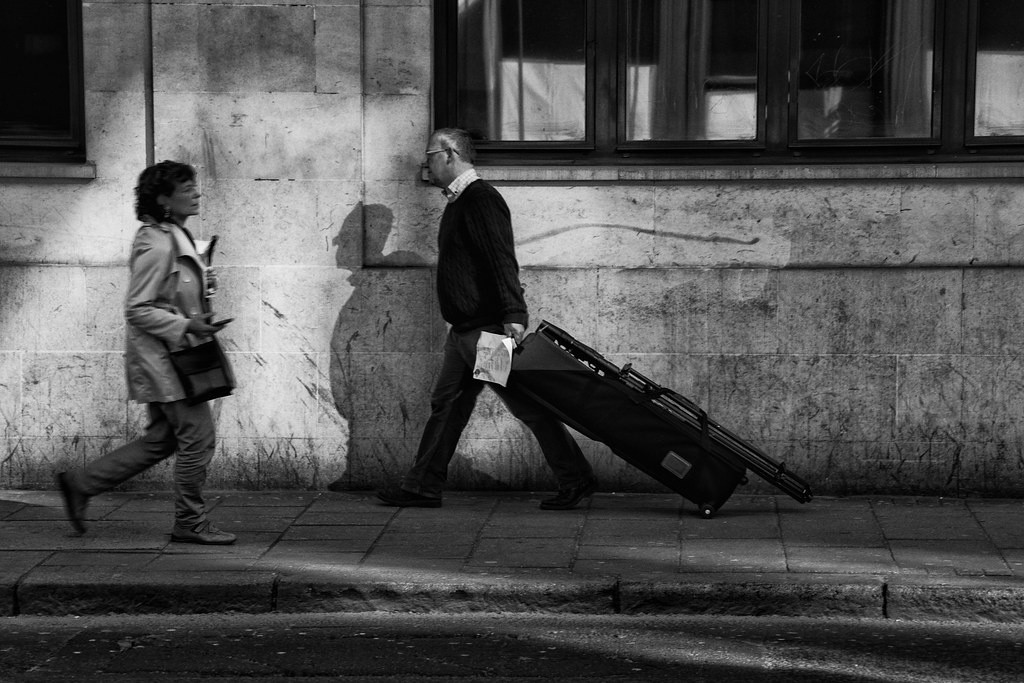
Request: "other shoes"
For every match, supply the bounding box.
[58,472,87,534]
[171,519,236,544]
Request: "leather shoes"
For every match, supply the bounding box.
[540,474,599,510]
[377,488,443,507]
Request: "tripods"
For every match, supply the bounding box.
[537,318,814,507]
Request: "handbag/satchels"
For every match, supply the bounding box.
[170,340,234,406]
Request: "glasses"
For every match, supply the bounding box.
[426,148,460,159]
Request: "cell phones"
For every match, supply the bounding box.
[210,317,234,325]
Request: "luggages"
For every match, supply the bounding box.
[506,331,746,519]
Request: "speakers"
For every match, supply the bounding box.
[509,332,749,516]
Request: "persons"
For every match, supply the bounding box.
[376,128,597,511]
[56,160,238,545]
